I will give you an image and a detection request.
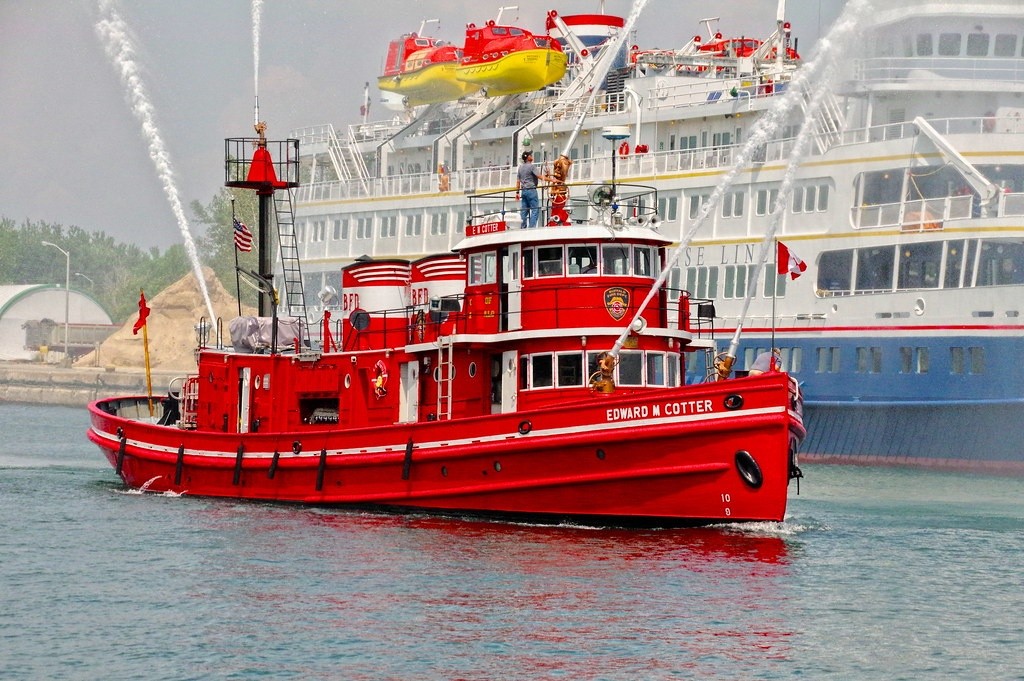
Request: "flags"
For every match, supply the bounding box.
[232,214,252,252]
[134,294,151,335]
[777,241,807,281]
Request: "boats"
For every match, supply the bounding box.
[455,5,570,99]
[375,18,482,108]
[87,113,809,529]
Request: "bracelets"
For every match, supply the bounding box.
[516,191,519,194]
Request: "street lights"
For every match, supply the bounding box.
[41,240,74,368]
[74,273,94,299]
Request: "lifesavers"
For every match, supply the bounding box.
[371,360,388,401]
[619,142,630,159]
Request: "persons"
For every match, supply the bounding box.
[748,347,782,378]
[516,150,559,228]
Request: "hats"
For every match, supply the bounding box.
[522,149,533,159]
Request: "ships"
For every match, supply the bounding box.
[272,1,1024,478]
